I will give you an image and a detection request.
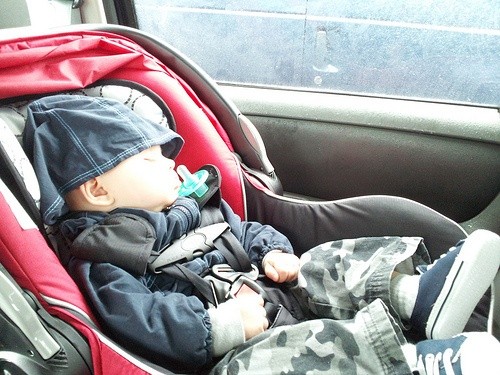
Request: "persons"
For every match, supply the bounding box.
[22,95,500,375]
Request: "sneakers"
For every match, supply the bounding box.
[416,333,500,373]
[410,229,499,343]
